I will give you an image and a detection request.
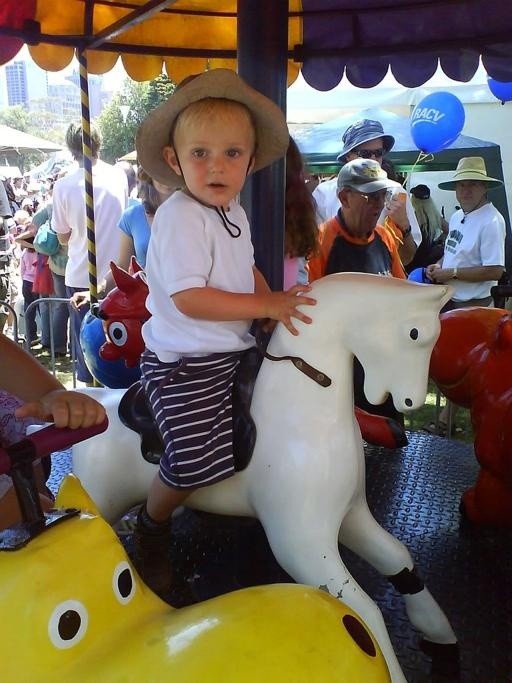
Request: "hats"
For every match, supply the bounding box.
[136,67,290,187]
[337,118,395,162]
[438,156,503,190]
[410,184,430,199]
[337,158,402,193]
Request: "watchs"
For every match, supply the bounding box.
[452,268,458,280]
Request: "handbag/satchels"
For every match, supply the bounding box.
[32,219,59,257]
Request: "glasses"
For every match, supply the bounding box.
[344,188,392,204]
[352,146,387,158]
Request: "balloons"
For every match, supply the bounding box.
[410,91,465,154]
[486,69,512,105]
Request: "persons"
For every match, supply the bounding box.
[134,68,316,599]
[0,331,105,537]
[0,117,177,390]
[285,120,449,280]
[425,157,506,433]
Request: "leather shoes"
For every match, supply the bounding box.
[132,504,185,606]
